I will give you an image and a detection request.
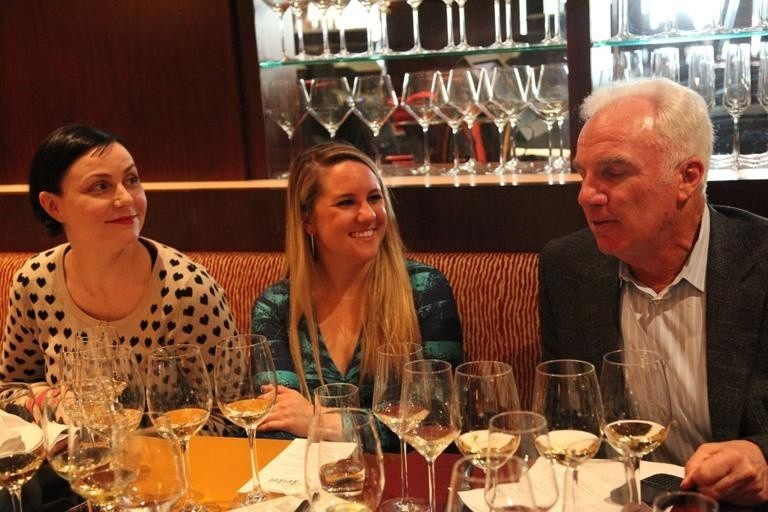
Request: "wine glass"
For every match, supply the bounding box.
[2,382,49,510]
[42,324,145,512]
[305,76,354,144]
[610,4,765,35]
[352,72,401,177]
[430,63,570,177]
[269,77,312,176]
[396,72,447,176]
[143,342,214,510]
[212,334,287,510]
[307,339,676,512]
[611,40,767,176]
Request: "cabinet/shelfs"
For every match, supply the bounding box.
[232,0,768,179]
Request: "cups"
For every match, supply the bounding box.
[652,486,720,512]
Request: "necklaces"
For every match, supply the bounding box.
[327,279,365,302]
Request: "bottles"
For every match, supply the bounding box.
[265,0,569,54]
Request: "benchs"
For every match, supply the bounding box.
[0,251,542,413]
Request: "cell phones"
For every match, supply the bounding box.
[611,474,684,507]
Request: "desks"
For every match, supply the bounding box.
[122,435,704,512]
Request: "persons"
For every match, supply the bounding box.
[537,77,767,509]
[252,142,467,454]
[0,125,246,439]
